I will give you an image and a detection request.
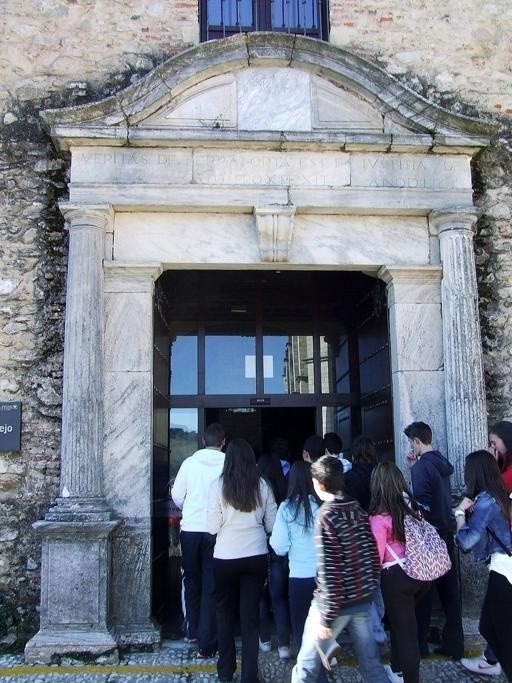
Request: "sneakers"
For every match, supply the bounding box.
[259,638,272,651]
[383,662,404,682]
[459,651,502,676]
[278,645,291,659]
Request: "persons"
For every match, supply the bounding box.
[452,447,509,681]
[294,431,391,655]
[403,419,467,661]
[268,456,339,668]
[205,434,280,682]
[360,458,429,683]
[254,447,297,661]
[291,453,394,683]
[460,417,510,679]
[171,423,236,643]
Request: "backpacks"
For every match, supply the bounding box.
[385,509,452,581]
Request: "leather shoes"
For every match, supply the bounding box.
[433,647,463,660]
